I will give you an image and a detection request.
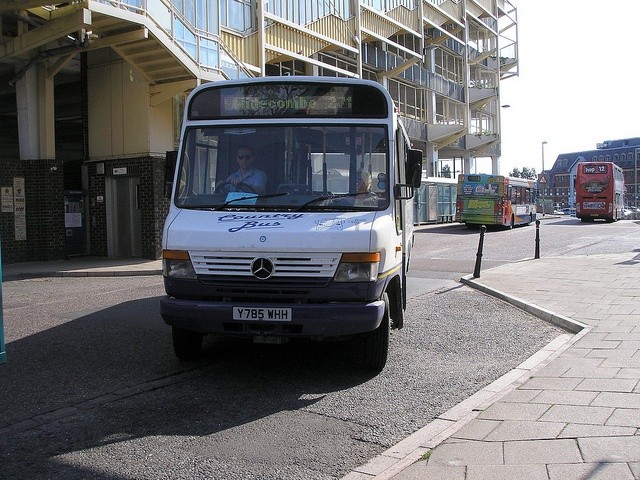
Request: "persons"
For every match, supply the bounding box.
[345,169,379,197]
[215,146,267,194]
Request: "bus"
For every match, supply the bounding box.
[158,75,423,377]
[454,173,538,230]
[575,161,625,222]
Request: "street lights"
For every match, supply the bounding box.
[542,141,548,217]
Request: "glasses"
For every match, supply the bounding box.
[237,153,250,160]
